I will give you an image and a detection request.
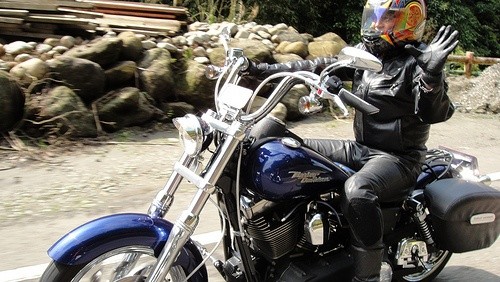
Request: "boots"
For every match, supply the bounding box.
[349,244,385,282]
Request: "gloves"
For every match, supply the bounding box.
[405,25,460,86]
[238,59,270,78]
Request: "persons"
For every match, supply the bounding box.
[233,0,460,281]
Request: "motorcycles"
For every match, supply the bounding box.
[39,29,500,282]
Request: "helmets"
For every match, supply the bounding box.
[360,0,425,56]
